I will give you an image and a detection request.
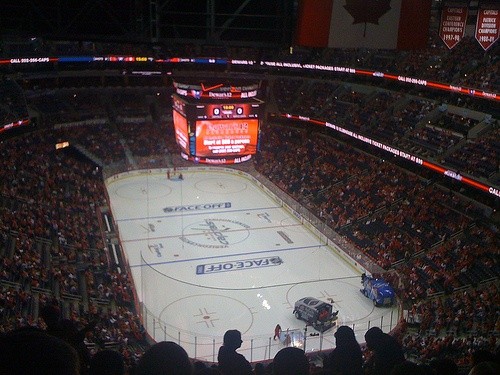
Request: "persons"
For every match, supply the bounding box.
[362,327,406,375]
[0,27,500,375]
[322,326,362,375]
[217,330,252,375]
[179,169,184,180]
[273,324,281,341]
[167,170,171,179]
[172,164,177,171]
